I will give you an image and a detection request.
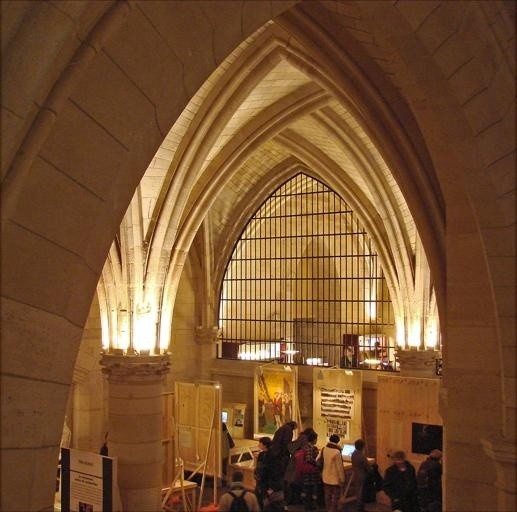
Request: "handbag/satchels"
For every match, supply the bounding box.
[294,450,319,474]
[316,458,323,470]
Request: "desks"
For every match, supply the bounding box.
[163,478,197,512]
[225,438,375,512]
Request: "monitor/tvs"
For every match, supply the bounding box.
[341,442,356,456]
[221,411,228,422]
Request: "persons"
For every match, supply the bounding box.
[216,470,261,511]
[412,448,441,511]
[339,343,358,370]
[248,421,348,511]
[349,437,378,511]
[222,422,231,486]
[381,450,416,512]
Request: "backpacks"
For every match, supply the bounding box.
[229,490,248,512]
